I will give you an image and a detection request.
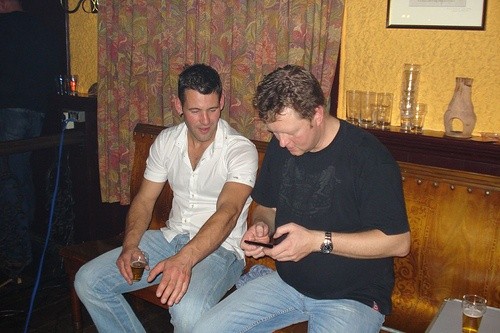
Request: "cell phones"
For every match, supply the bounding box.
[244,239,273,248]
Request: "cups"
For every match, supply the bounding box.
[370,104,389,129]
[66,75,78,96]
[462,294,487,333]
[377,93,394,127]
[399,62,427,132]
[346,90,362,124]
[358,91,376,125]
[128,250,150,282]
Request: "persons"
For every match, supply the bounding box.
[190,65,412,333]
[0,0,67,319]
[74,65,258,333]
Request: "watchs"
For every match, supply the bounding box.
[320,231,333,254]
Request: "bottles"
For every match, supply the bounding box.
[56,73,66,96]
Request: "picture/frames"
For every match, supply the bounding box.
[384,0,488,30]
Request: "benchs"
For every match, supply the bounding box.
[61,125,500,333]
